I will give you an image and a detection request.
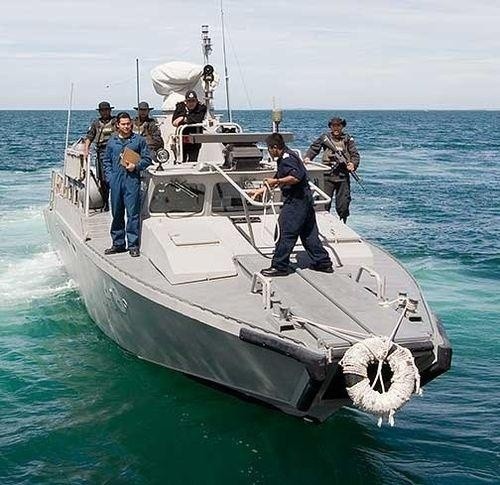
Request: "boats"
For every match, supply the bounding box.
[42,0,455,432]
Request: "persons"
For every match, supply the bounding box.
[102,110,152,257]
[83,102,120,213]
[250,132,335,276]
[131,100,163,150]
[305,116,360,222]
[171,91,207,162]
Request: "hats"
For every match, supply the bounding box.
[133,102,154,110]
[328,118,346,127]
[185,90,197,103]
[96,102,113,110]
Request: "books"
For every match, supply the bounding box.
[120,147,141,169]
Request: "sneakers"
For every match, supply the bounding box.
[105,244,125,254]
[130,249,140,257]
[101,203,108,212]
[260,267,288,277]
[314,266,332,273]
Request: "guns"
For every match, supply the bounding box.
[320,135,368,193]
